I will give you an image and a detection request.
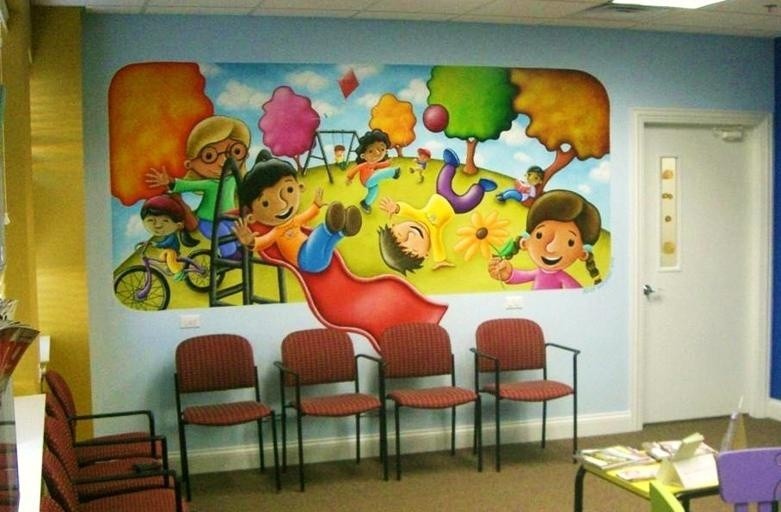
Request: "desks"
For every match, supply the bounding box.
[569,435,733,511]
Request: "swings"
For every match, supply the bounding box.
[330,131,346,167]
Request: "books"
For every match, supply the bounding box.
[572,431,719,491]
[0,296,42,413]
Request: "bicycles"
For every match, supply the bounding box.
[112,240,226,309]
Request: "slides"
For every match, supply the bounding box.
[222,208,449,358]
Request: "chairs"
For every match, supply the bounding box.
[647,481,686,512]
[37,366,190,512]
[378,320,484,479]
[274,327,387,490]
[711,444,781,510]
[463,320,591,473]
[173,332,284,502]
